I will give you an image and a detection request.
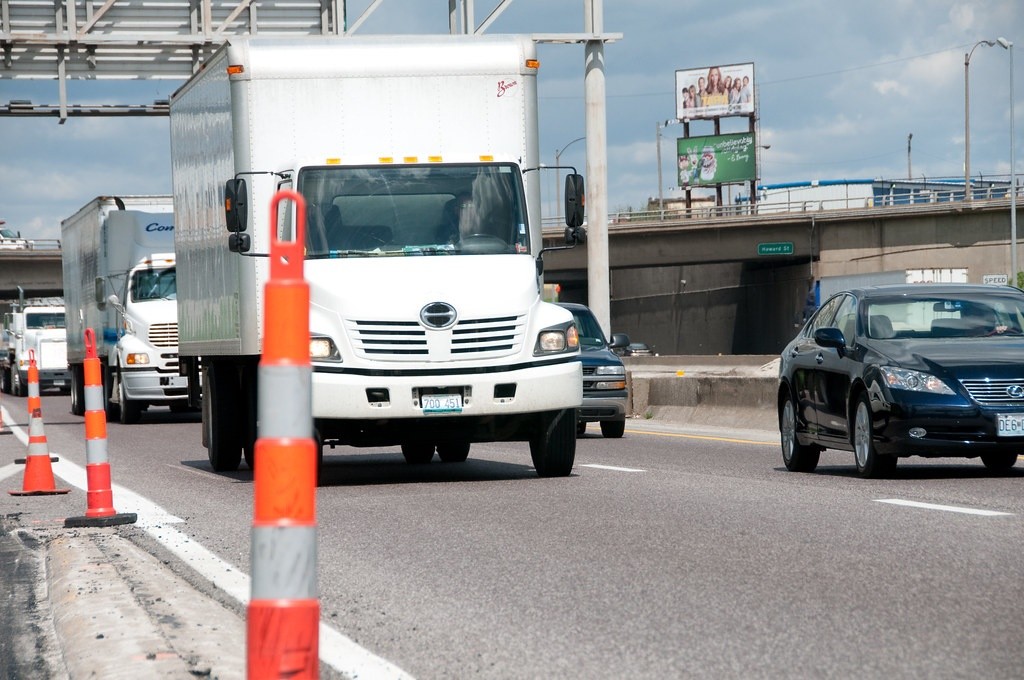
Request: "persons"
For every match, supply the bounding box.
[681,66,751,109]
[973,310,1021,335]
[436,192,511,245]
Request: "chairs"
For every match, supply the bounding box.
[844,316,894,350]
[929,318,971,337]
[442,200,462,242]
[306,202,392,253]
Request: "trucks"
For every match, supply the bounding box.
[0,286,72,397]
[60,193,203,426]
[168,30,588,477]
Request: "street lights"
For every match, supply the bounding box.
[996,39,1019,289]
[961,39,999,202]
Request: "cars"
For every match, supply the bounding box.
[623,342,655,357]
[0,228,34,249]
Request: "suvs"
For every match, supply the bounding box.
[549,302,631,440]
[774,283,1024,479]
